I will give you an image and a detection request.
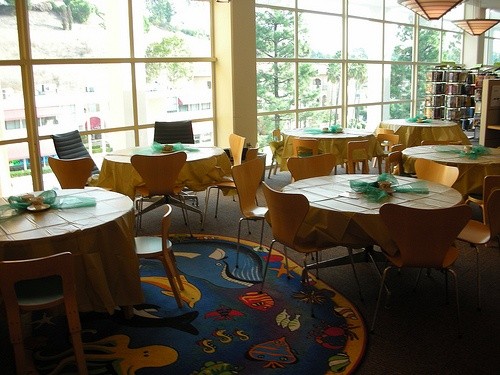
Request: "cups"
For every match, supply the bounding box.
[22,195,43,208]
[164,145,173,151]
[336,129,343,132]
[323,128,328,131]
[463,145,473,152]
[417,118,423,121]
[378,180,391,191]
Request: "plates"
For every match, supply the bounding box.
[387,190,396,194]
[160,150,175,153]
[416,120,427,122]
[27,203,50,211]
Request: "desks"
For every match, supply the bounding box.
[402,145,500,203]
[95,142,230,220]
[281,175,463,299]
[374,119,472,149]
[270,129,377,176]
[0,189,134,311]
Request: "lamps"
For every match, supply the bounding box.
[396,0,472,21]
[451,0,500,37]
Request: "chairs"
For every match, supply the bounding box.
[133,151,203,236]
[153,120,196,144]
[53,131,98,175]
[134,204,184,307]
[203,131,500,332]
[46,158,91,188]
[0,251,87,375]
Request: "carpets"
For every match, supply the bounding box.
[0,231,365,375]
